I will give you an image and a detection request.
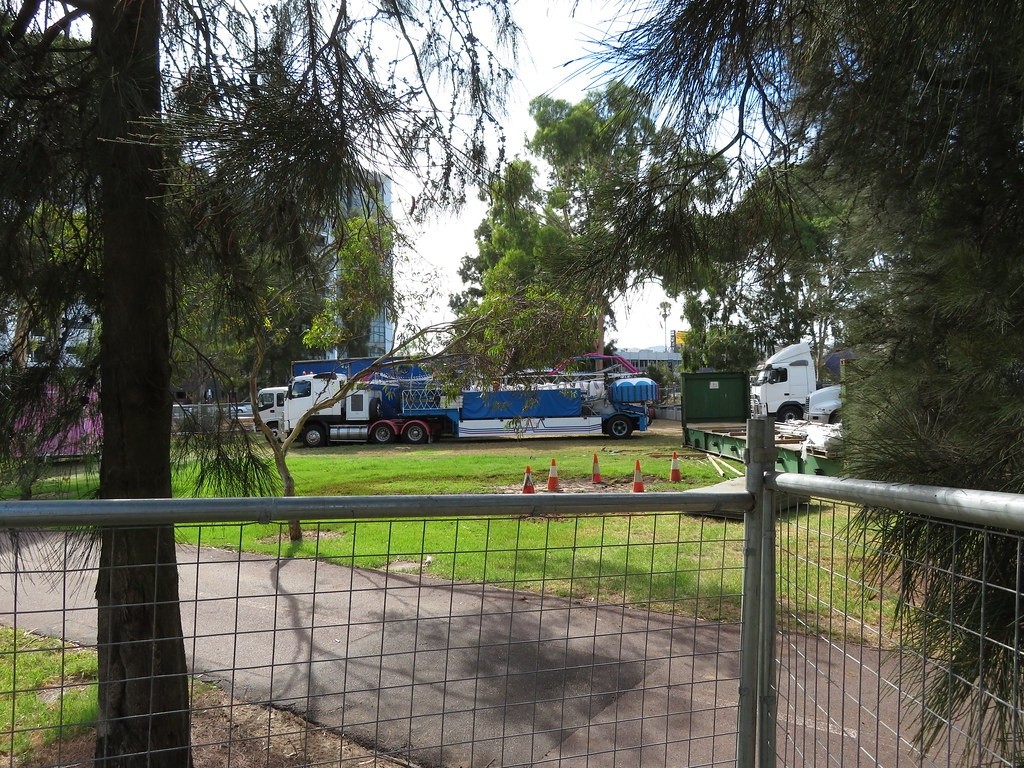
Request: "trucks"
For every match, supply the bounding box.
[802,382,842,424]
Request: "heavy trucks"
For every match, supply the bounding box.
[251,386,291,444]
[751,341,850,423]
[282,370,659,447]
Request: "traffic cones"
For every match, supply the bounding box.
[542,458,564,491]
[631,459,646,492]
[589,453,602,483]
[669,451,681,482]
[516,465,537,494]
